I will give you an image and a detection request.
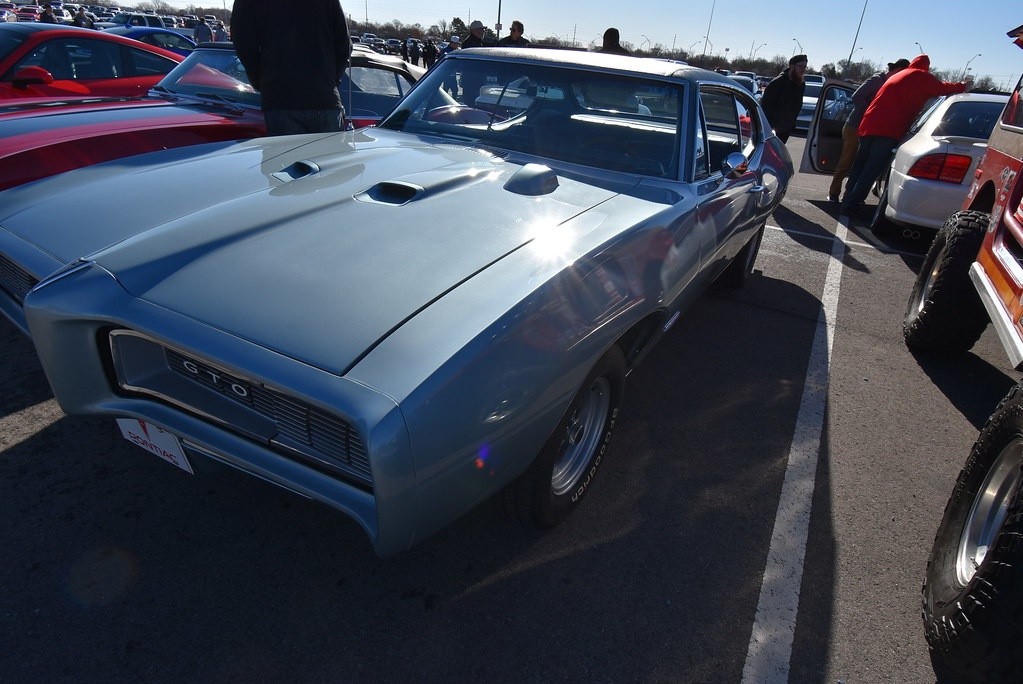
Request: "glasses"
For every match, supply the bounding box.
[510,28,516,31]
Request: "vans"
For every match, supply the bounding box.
[635,57,689,98]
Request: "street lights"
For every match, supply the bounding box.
[702,36,713,55]
[690,41,701,52]
[793,39,803,55]
[962,54,981,81]
[642,35,650,49]
[753,44,767,60]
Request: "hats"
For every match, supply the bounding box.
[888,59,911,70]
[788,55,806,65]
[470,21,487,29]
[451,36,459,42]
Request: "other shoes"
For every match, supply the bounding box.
[827,196,835,202]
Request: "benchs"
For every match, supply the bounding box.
[518,112,751,172]
[135,67,161,76]
[341,89,400,116]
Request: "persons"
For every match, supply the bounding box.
[829,59,910,207]
[401,40,410,63]
[839,55,973,217]
[40,4,58,23]
[422,39,438,70]
[438,36,461,100]
[597,27,639,113]
[194,17,213,43]
[409,41,422,65]
[74,7,98,29]
[230,0,354,136]
[214,22,227,42]
[459,21,487,108]
[496,20,530,87]
[762,54,808,145]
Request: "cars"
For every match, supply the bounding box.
[795,82,847,130]
[0,41,495,192]
[474,74,652,118]
[1,48,793,557]
[700,69,776,121]
[798,77,1012,239]
[0,22,257,99]
[65,24,236,73]
[901,26,1022,684]
[0,1,227,46]
[351,33,449,55]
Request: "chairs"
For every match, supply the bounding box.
[91,45,118,79]
[40,43,76,80]
